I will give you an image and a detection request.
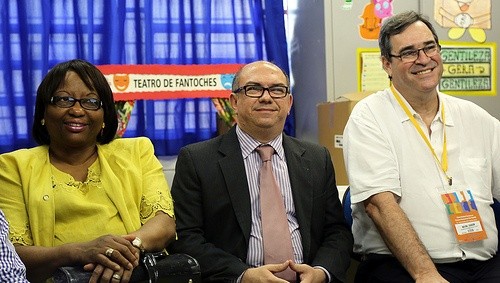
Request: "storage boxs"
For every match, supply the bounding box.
[317,91,374,186]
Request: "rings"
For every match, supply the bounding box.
[105,249,114,258]
[113,274,121,280]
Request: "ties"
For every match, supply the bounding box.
[257,145,297,281]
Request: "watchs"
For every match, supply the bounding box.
[132,237,146,254]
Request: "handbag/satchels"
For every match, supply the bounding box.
[49,252,201,283]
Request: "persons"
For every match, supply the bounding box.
[0,59,178,283]
[172,60,355,283]
[341,12,500,283]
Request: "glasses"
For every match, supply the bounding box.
[48,96,102,111]
[234,85,290,99]
[390,44,441,63]
